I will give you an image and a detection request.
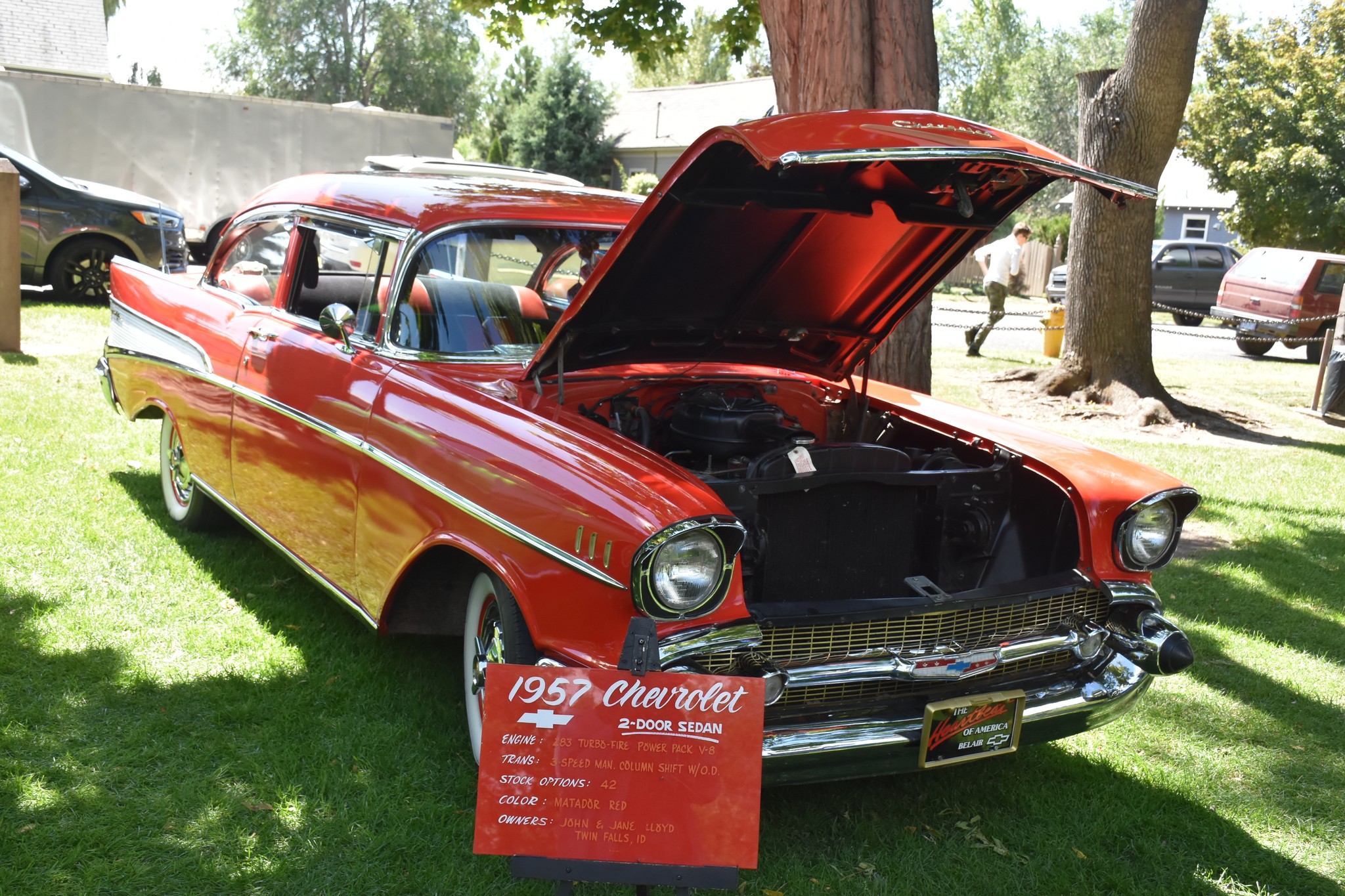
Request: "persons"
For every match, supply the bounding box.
[965,221,1034,357]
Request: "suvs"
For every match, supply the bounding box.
[1210,247,1345,362]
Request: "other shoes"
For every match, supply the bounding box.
[966,344,985,357]
[965,326,976,346]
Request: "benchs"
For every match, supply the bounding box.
[269,275,547,322]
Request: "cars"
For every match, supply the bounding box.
[0,146,189,302]
[98,108,1193,880]
[1044,239,1244,327]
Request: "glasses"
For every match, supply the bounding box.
[1025,236,1030,241]
